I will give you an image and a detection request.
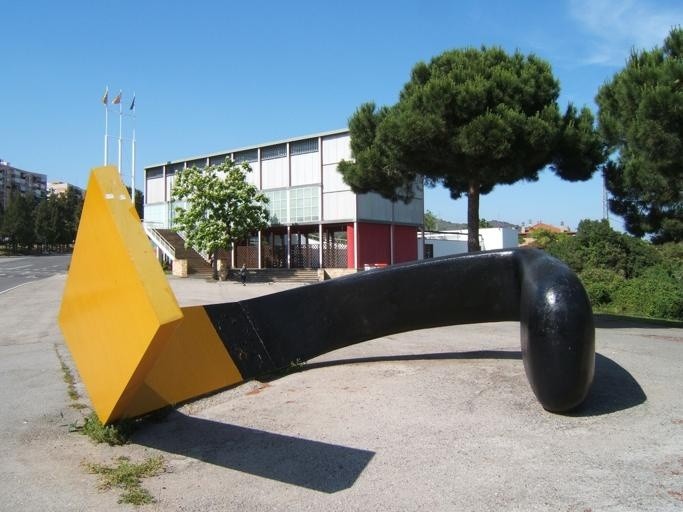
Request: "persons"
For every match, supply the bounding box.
[240,263,249,286]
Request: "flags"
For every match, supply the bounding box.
[112,92,121,104]
[129,96,135,110]
[102,87,108,105]
